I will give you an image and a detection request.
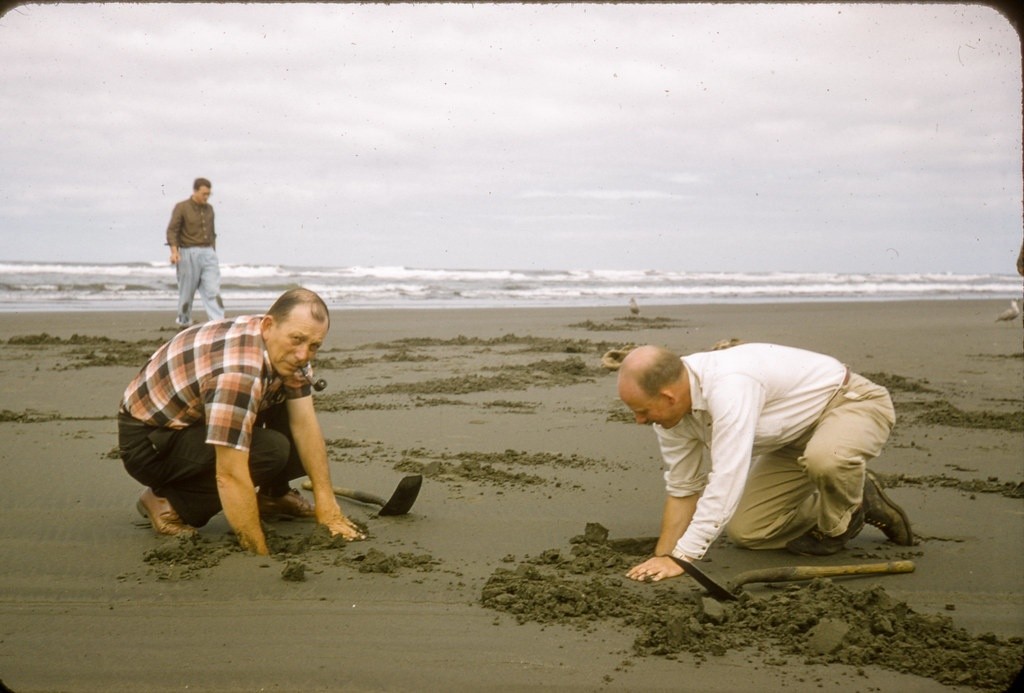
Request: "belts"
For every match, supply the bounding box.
[843,367,850,385]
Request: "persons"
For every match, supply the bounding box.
[618,343,913,582]
[118,288,366,557]
[166,178,225,333]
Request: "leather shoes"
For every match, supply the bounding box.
[256,488,318,523]
[137,487,200,541]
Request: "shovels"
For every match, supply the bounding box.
[663,551,918,602]
[300,473,426,519]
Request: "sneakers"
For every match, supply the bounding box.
[863,471,914,546]
[786,508,865,558]
